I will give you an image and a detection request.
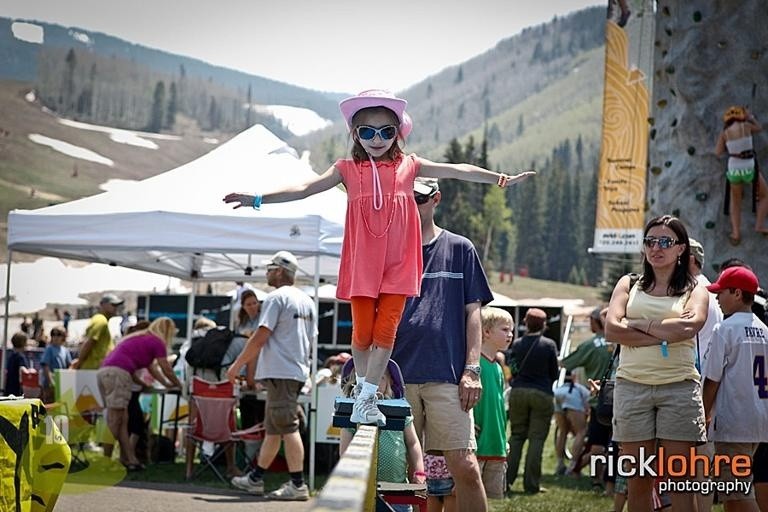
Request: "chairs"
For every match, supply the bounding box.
[185,395,241,488]
[67,440,88,472]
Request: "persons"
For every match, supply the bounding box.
[118,321,151,462]
[2,332,38,396]
[231,279,256,334]
[224,250,319,502]
[496,351,515,425]
[236,289,263,380]
[55,307,63,320]
[94,316,183,473]
[716,105,768,240]
[207,284,212,295]
[387,174,495,512]
[472,306,515,501]
[494,308,560,494]
[165,350,180,369]
[652,237,724,512]
[62,311,73,337]
[604,212,711,512]
[222,88,537,426]
[164,319,218,450]
[21,316,32,336]
[614,420,662,512]
[599,308,619,500]
[152,287,157,294]
[552,380,593,480]
[38,326,74,404]
[338,355,428,512]
[184,327,263,482]
[420,429,457,512]
[31,311,44,339]
[554,309,614,482]
[71,294,126,453]
[119,311,132,336]
[165,286,171,295]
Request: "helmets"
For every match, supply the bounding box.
[723,106,744,122]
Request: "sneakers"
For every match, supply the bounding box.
[269,481,310,501]
[565,469,582,478]
[354,385,362,401]
[351,400,386,426]
[553,464,568,476]
[232,472,264,495]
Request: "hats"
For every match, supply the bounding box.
[413,176,438,195]
[341,91,412,140]
[706,267,758,293]
[100,294,124,305]
[587,307,600,320]
[262,251,297,274]
[525,308,546,324]
[689,238,704,269]
[342,356,406,401]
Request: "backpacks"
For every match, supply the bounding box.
[184,326,250,382]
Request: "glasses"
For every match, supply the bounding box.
[643,235,681,249]
[415,195,434,205]
[353,125,399,140]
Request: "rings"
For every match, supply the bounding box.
[474,397,479,399]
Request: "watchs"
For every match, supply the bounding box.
[464,364,481,376]
[253,193,263,212]
[497,173,507,189]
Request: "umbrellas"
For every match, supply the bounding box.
[0,123,348,498]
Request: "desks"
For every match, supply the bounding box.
[1,397,71,512]
[136,386,181,463]
[238,389,309,473]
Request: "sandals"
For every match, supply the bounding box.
[127,463,145,471]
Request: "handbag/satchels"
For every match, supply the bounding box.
[597,273,640,426]
